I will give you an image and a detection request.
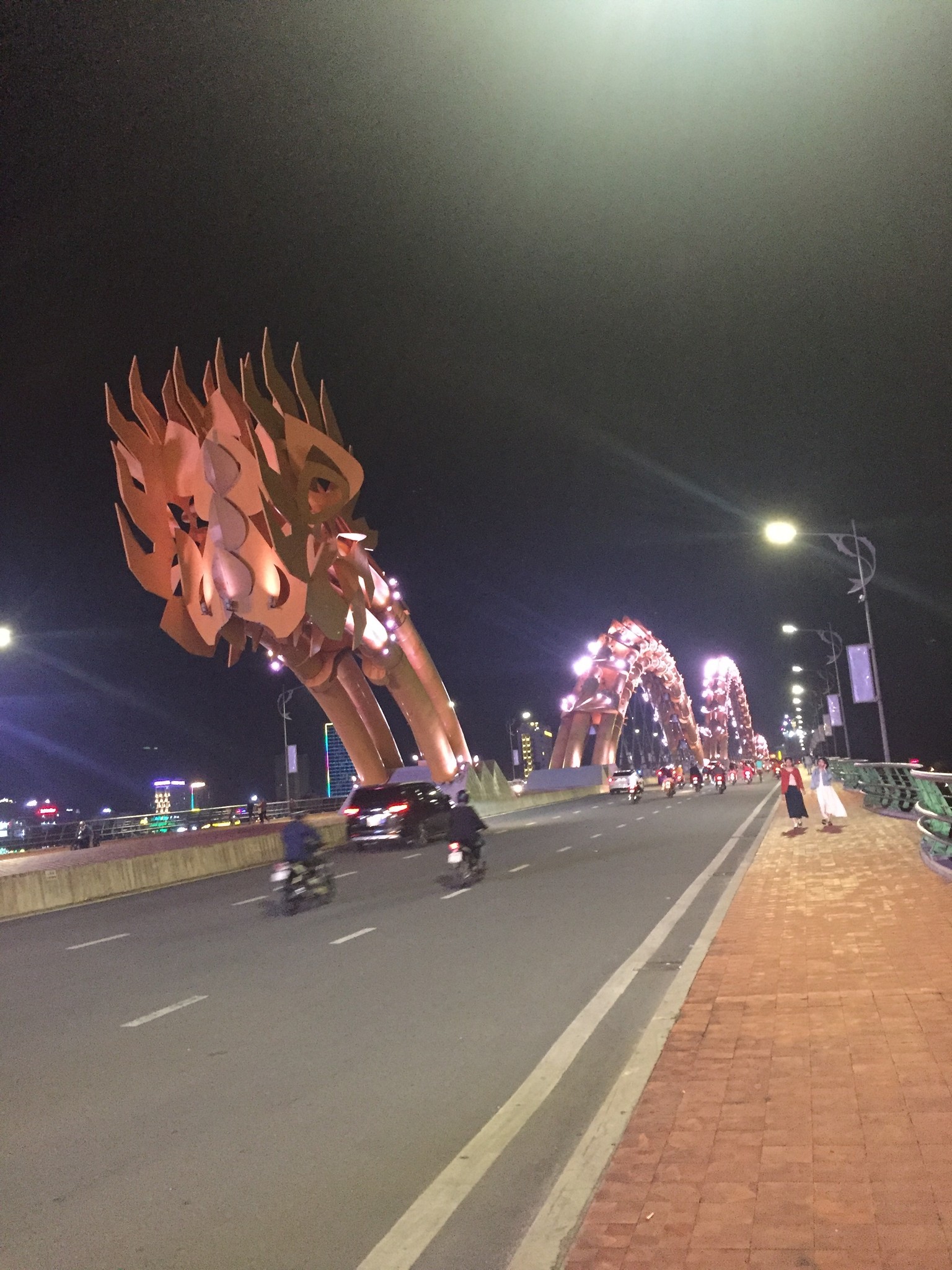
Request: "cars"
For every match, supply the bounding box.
[608,769,645,794]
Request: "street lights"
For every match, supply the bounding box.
[763,519,891,762]
[510,712,531,780]
[190,782,205,809]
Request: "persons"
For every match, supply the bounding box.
[78,820,94,848]
[775,756,809,828]
[447,790,488,870]
[741,758,782,782]
[289,797,300,820]
[660,765,684,791]
[689,764,703,788]
[713,763,726,789]
[281,813,329,895]
[629,770,640,794]
[247,797,269,823]
[728,760,738,781]
[811,757,848,826]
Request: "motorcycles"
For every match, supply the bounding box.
[691,768,738,794]
[627,781,644,805]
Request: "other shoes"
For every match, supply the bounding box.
[794,827,798,830]
[828,821,832,826]
[798,821,802,827]
[822,819,826,824]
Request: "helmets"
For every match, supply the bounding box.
[458,790,469,803]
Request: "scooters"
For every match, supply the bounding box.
[657,772,687,798]
[71,832,100,851]
[743,763,782,784]
[448,825,490,888]
[269,839,337,916]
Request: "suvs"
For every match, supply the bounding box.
[345,782,458,852]
